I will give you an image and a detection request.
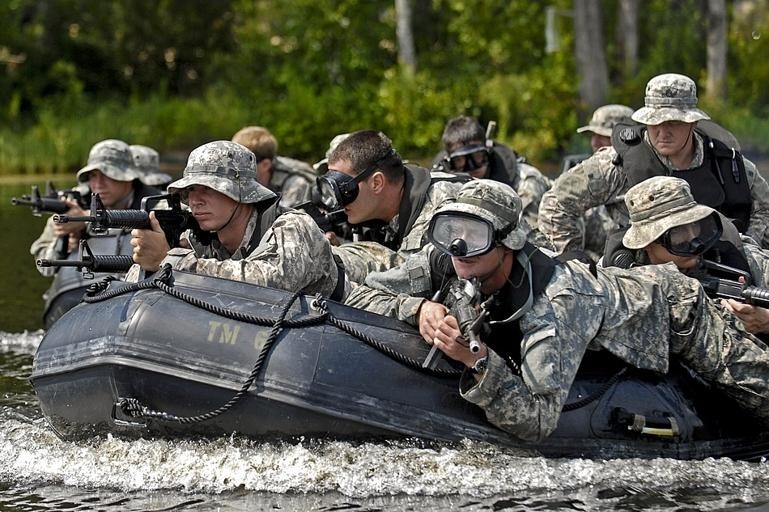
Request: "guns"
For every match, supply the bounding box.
[292,200,348,240]
[43,178,84,201]
[54,191,211,250]
[35,238,157,282]
[11,185,77,215]
[615,253,769,307]
[422,256,491,370]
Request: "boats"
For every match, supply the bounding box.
[30,272,765,459]
[40,228,173,327]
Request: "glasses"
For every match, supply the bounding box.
[316,169,363,211]
[424,209,504,257]
[437,144,490,172]
[666,212,723,257]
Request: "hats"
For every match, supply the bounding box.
[311,132,351,169]
[129,144,173,186]
[76,138,143,185]
[630,72,712,126]
[433,178,527,251]
[166,140,278,206]
[621,175,717,250]
[576,103,639,138]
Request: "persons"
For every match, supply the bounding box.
[31,74,769,443]
